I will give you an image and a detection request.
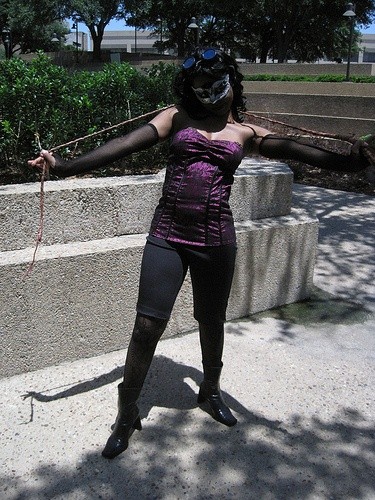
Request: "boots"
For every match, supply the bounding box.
[197,361,237,426]
[103,383,143,459]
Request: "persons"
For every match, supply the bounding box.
[29,49,375,459]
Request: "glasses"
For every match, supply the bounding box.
[182,49,217,71]
